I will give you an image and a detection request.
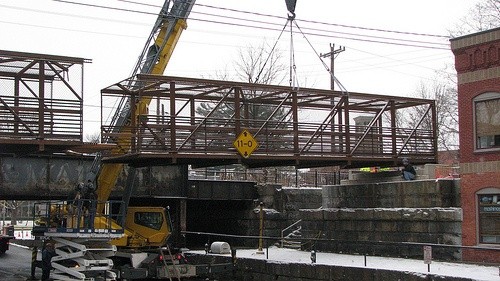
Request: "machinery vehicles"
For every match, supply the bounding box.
[36,0,298,243]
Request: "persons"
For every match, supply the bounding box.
[402,156,417,182]
[83,182,97,232]
[72,182,85,210]
[40,236,54,281]
